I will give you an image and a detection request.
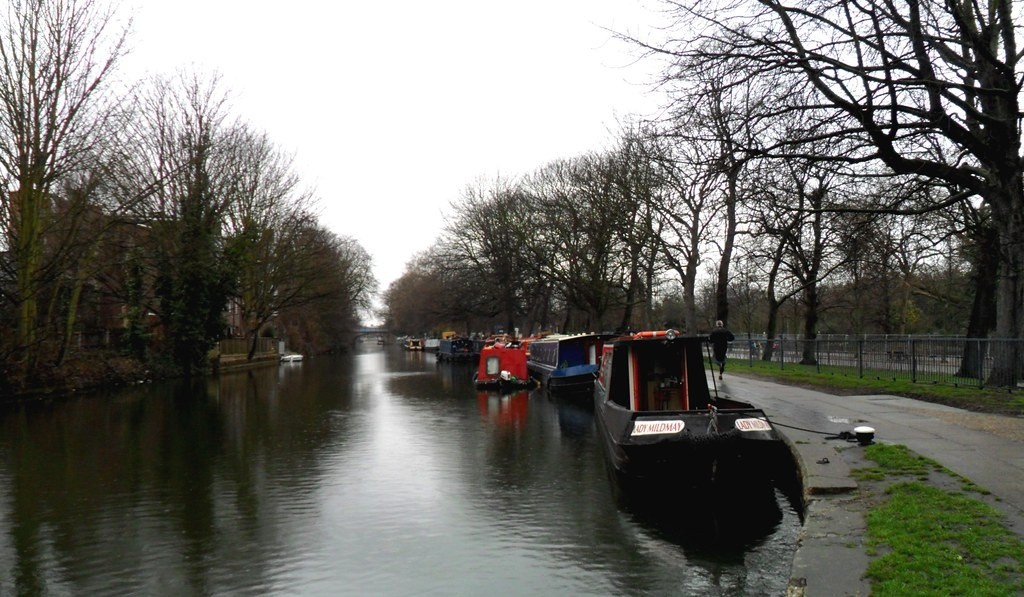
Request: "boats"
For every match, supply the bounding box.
[280,353,304,363]
[473,332,555,400]
[592,328,801,492]
[526,332,625,394]
[376,334,394,346]
[396,331,486,359]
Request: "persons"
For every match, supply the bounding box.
[709,320,735,379]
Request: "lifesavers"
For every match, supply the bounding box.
[634,329,680,339]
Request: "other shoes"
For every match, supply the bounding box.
[719,375,723,380]
[720,363,725,374]
